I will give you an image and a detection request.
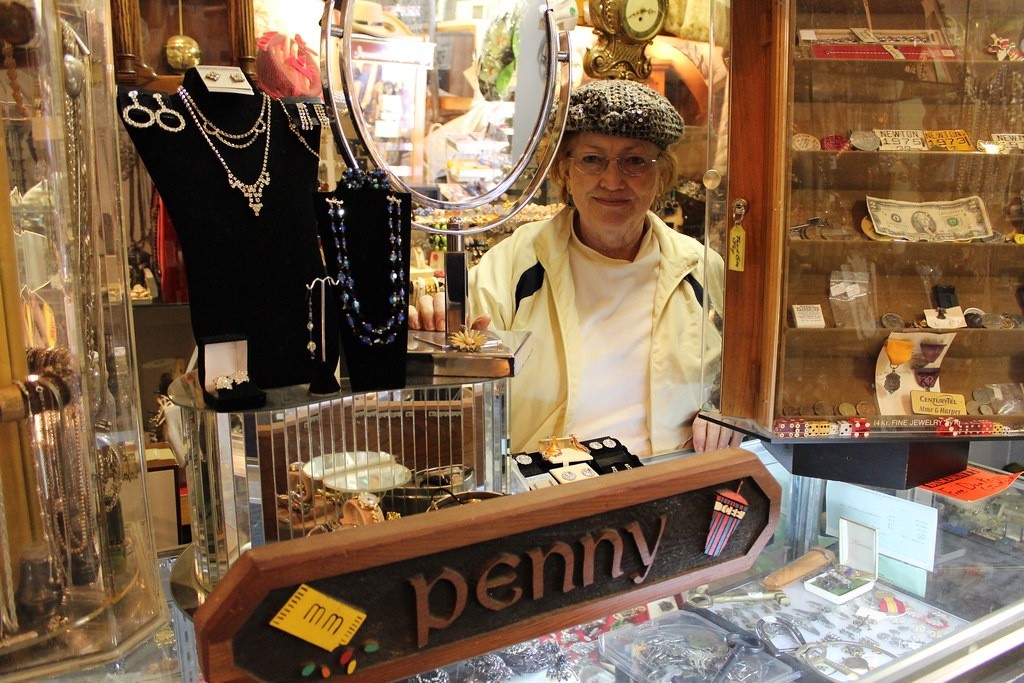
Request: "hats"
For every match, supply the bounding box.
[547,79,684,149]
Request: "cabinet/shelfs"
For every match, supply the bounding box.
[723,0,1024,426]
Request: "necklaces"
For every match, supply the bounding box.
[328,194,408,344]
[179,82,270,219]
[7,0,123,597]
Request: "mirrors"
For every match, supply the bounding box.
[320,0,572,376]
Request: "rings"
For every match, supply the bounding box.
[702,402,714,410]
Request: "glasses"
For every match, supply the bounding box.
[565,152,660,175]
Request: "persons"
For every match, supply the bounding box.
[409,81,752,470]
